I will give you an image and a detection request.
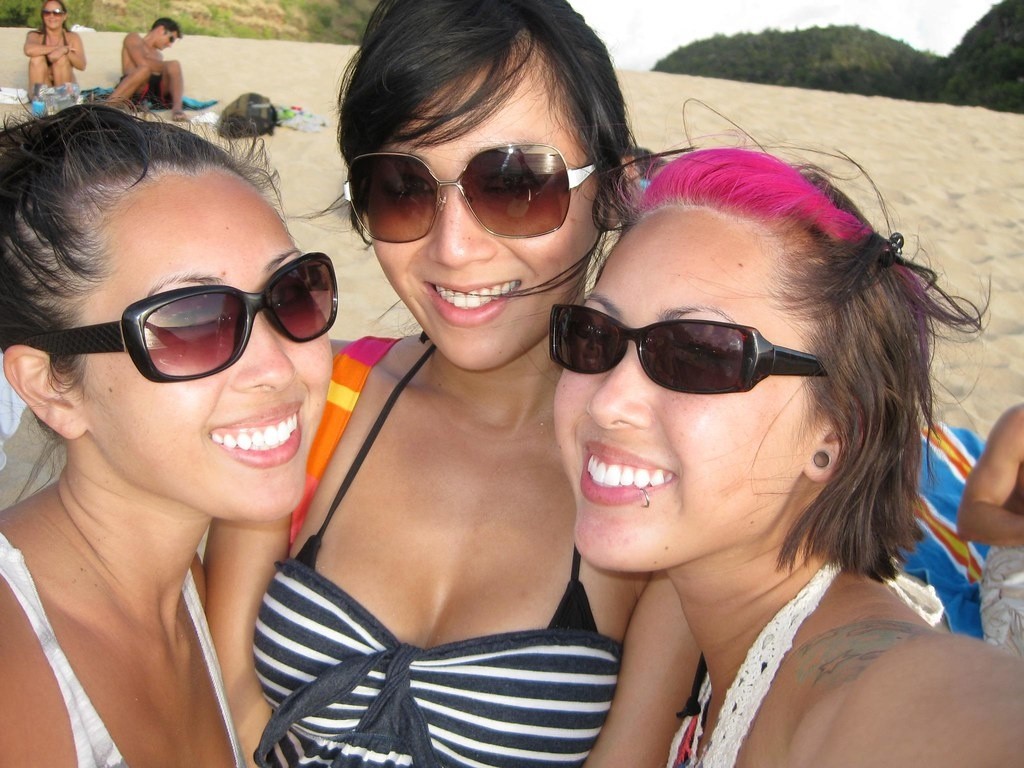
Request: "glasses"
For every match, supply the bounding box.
[343,143,597,243]
[169,35,174,41]
[549,304,827,395]
[27,253,337,383]
[42,8,66,16]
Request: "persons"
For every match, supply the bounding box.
[547,97,1024,765]
[101,17,187,121]
[957,403,1024,659]
[0,89,333,768]
[205,0,703,768]
[24,1,87,98]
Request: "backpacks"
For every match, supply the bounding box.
[218,92,282,138]
